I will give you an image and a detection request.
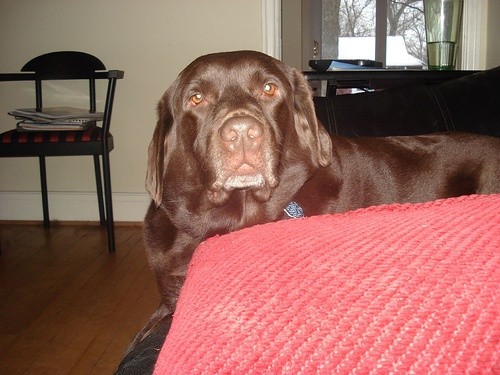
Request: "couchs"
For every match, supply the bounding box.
[152,192,500,374]
[114,66,500,375]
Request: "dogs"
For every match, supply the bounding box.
[141,50,500,324]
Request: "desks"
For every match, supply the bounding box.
[302,70,467,96]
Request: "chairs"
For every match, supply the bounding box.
[0,50,124,254]
